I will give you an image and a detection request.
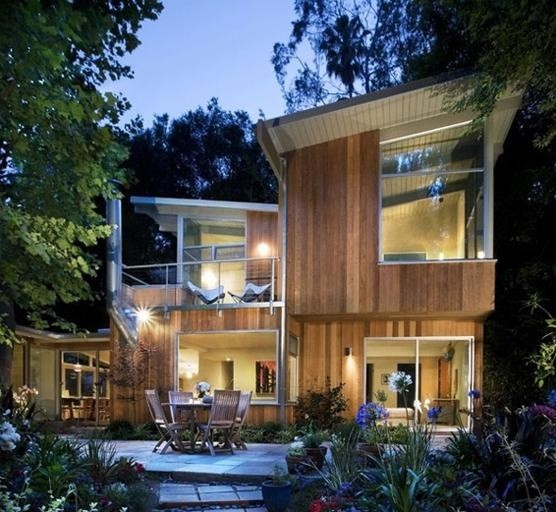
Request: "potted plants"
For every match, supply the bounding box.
[293,374,350,469]
[373,390,389,408]
[262,464,292,511]
[286,446,309,473]
[358,427,383,466]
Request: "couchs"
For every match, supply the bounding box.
[374,407,414,432]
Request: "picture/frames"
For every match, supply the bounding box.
[381,373,390,384]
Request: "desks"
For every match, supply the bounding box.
[432,400,456,425]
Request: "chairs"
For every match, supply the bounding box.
[143,389,252,456]
[228,282,271,302]
[58,396,111,426]
[187,280,225,304]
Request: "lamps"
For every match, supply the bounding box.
[256,242,271,257]
[345,348,351,356]
